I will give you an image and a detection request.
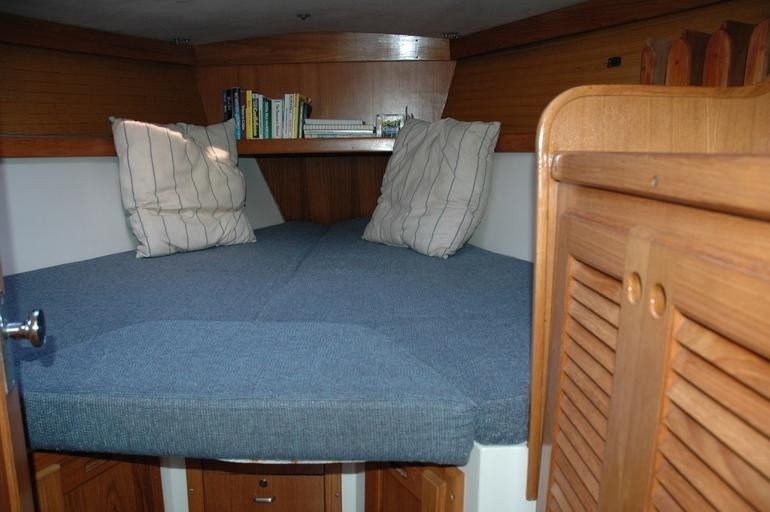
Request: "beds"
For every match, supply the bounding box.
[0,148,538,466]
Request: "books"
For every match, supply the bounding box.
[219,87,376,137]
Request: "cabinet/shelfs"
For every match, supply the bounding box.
[524,74,770,512]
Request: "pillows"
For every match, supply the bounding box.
[103,113,257,259]
[360,118,502,261]
